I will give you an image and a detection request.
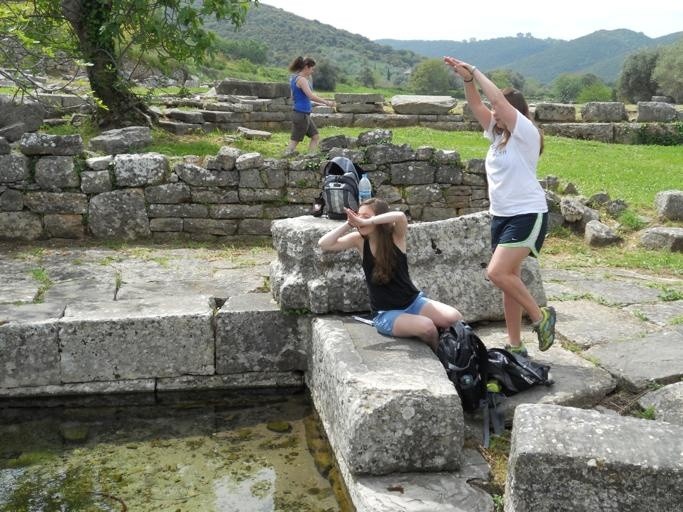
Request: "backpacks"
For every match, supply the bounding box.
[312,156,374,221]
[436,320,550,412]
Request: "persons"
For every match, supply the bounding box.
[285,55,336,151]
[440,55,556,358]
[301,57,313,90]
[316,197,462,354]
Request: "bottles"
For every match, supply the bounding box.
[358,173,371,207]
[460,375,473,386]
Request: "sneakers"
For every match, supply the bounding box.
[532,306,556,351]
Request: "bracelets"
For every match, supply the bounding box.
[463,76,473,83]
[370,214,376,226]
[344,220,355,229]
[470,65,476,75]
